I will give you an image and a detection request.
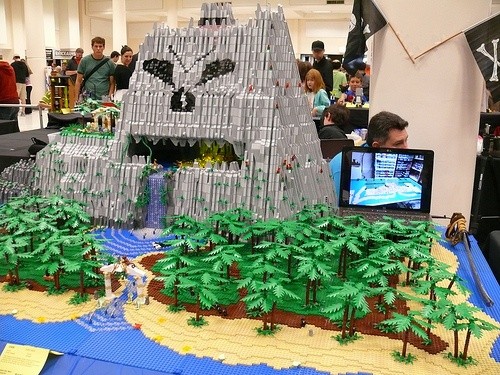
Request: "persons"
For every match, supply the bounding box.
[295,41,369,140]
[0,59,20,135]
[0,36,139,121]
[328,111,410,208]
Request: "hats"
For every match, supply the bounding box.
[311,41,324,51]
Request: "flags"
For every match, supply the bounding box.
[342,0,389,67]
[462,12,500,105]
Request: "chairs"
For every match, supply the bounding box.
[320,138,354,159]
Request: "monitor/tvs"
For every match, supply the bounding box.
[339,146,434,213]
